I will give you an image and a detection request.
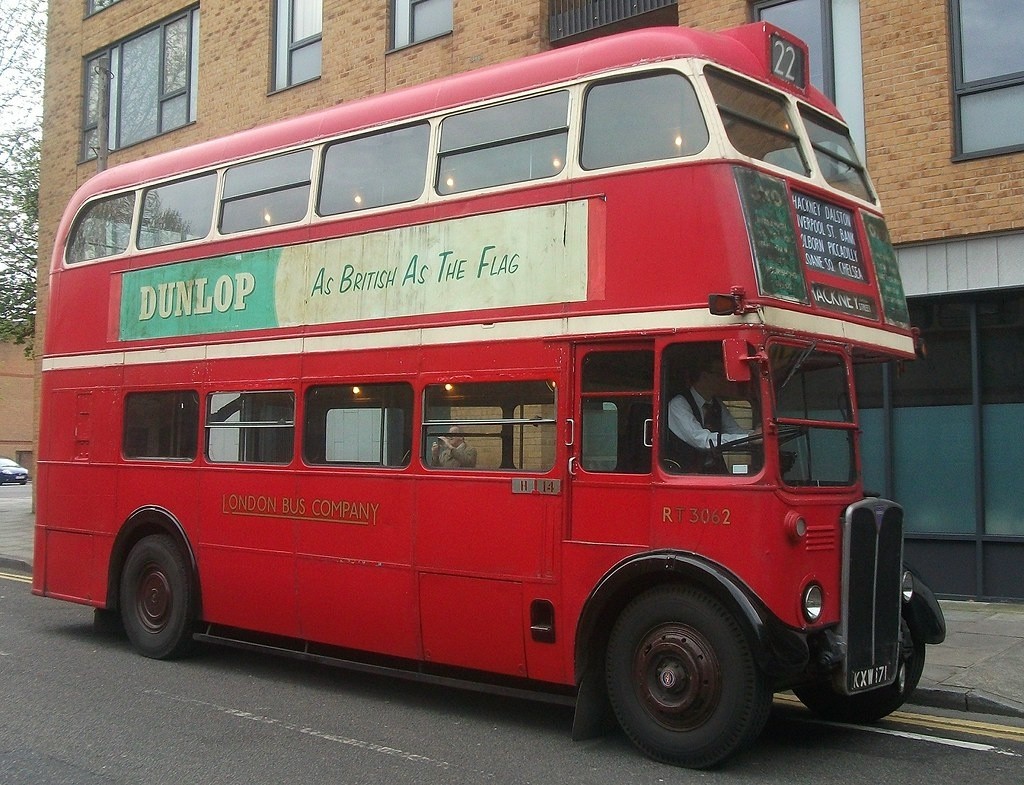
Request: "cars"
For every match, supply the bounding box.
[0,455,29,486]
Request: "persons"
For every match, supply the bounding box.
[666,349,763,474]
[431,425,477,468]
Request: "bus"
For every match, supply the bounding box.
[31,19,947,771]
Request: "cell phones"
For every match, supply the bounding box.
[438,438,442,444]
